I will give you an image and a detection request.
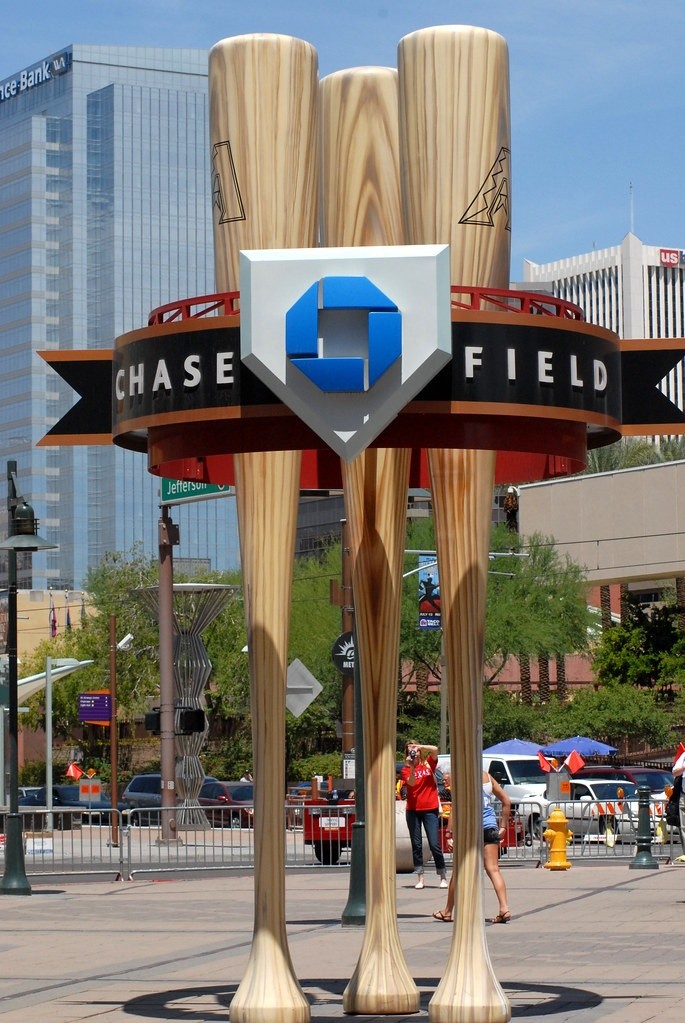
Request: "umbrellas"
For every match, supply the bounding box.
[538,734,619,758]
[481,737,546,756]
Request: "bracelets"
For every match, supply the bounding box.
[500,827,506,829]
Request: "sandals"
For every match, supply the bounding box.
[492,912,510,923]
[433,912,451,922]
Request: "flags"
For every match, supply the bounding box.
[564,749,585,774]
[67,606,71,634]
[81,604,87,627]
[51,594,57,637]
[538,752,551,772]
[65,762,84,780]
[673,743,685,765]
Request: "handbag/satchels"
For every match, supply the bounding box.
[665,775,683,826]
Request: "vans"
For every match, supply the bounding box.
[121,774,219,826]
[571,765,685,811]
[437,753,573,839]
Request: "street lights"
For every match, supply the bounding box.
[0,459,59,902]
[45,656,80,832]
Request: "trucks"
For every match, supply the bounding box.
[302,762,456,863]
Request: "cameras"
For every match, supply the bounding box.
[410,750,417,760]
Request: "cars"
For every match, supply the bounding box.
[197,781,300,828]
[19,784,134,832]
[518,778,680,846]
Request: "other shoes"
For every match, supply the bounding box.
[415,883,424,889]
[440,879,448,890]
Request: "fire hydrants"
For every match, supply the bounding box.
[541,807,574,871]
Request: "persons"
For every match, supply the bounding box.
[432,770,512,923]
[240,769,253,782]
[401,739,448,889]
[438,773,452,828]
[672,752,685,862]
[396,770,403,800]
